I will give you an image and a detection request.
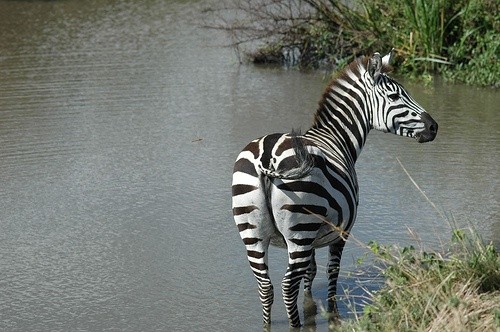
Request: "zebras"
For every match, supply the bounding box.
[231,47,439,330]
